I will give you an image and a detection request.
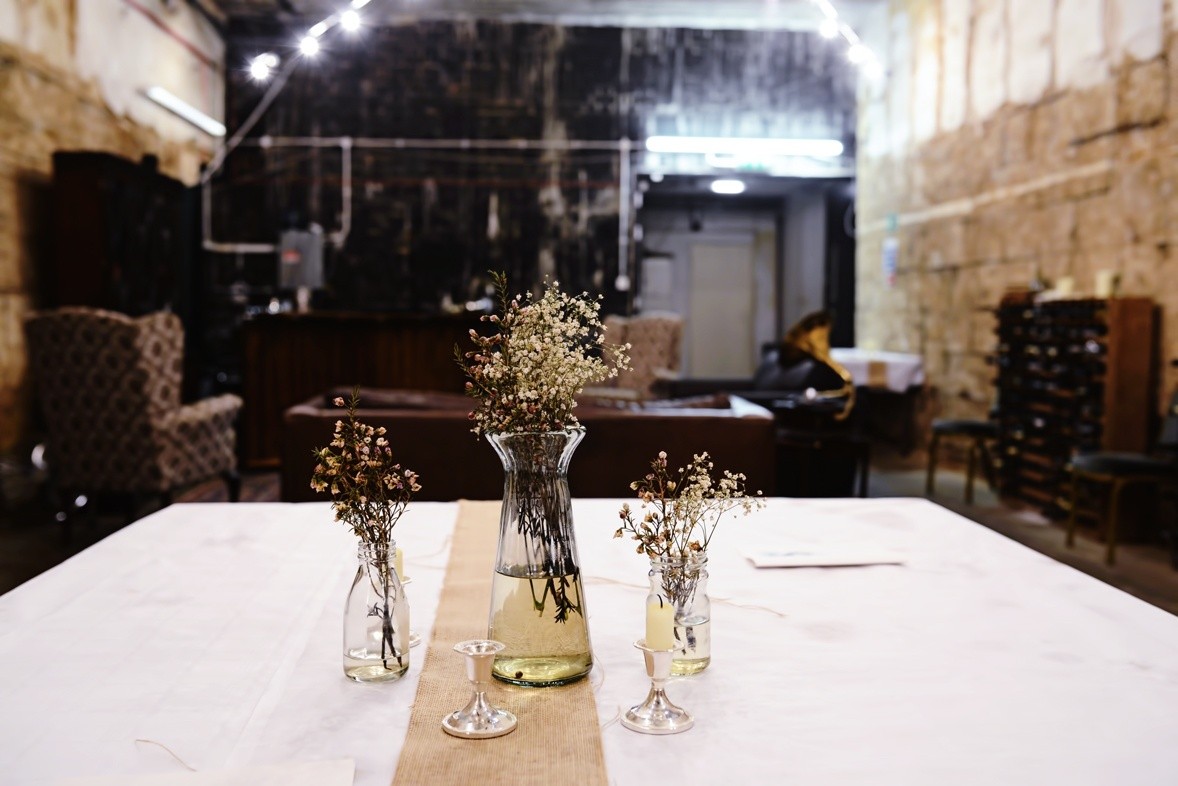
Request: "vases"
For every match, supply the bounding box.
[343,539,411,682]
[485,430,595,689]
[649,550,714,676]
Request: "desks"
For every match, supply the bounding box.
[828,347,925,456]
[0,496,1178,786]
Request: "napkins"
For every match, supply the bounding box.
[744,528,907,568]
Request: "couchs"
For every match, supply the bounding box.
[281,388,775,503]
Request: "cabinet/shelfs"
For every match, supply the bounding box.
[989,290,1154,543]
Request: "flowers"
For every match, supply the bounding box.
[310,389,422,671]
[613,451,766,656]
[452,270,635,624]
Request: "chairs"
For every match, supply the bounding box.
[17,309,244,523]
[924,403,998,503]
[1063,388,1178,567]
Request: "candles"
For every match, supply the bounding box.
[647,595,675,650]
